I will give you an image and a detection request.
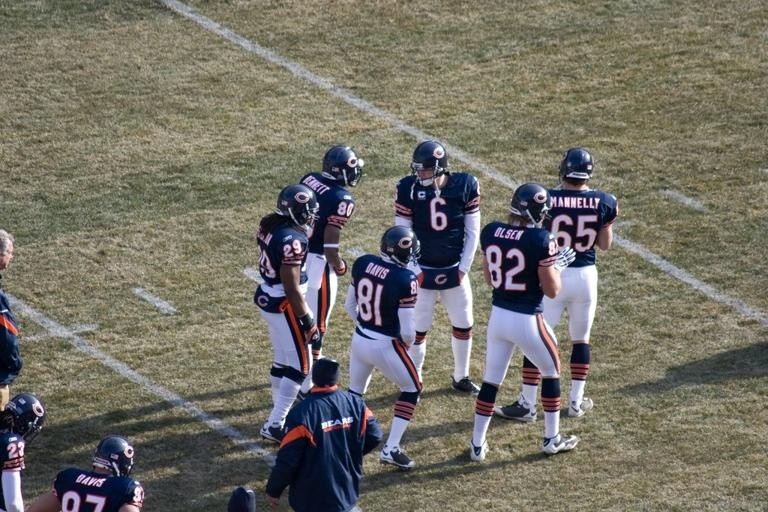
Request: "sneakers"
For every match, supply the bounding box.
[494,394,539,422]
[471,439,488,461]
[260,418,287,445]
[543,433,577,454]
[451,375,481,396]
[380,442,416,468]
[568,398,593,417]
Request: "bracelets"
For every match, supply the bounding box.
[296,313,314,332]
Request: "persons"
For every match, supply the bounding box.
[490,149,620,424]
[253,183,321,444]
[0,230,22,411]
[299,144,363,398]
[0,395,46,510]
[345,226,422,467]
[394,140,481,396]
[471,183,577,461]
[28,437,144,512]
[264,357,384,512]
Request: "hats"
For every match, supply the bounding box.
[228,487,256,512]
[311,359,339,386]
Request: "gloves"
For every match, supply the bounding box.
[554,246,576,272]
[305,320,321,344]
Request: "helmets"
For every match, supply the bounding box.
[560,148,595,178]
[93,437,135,477]
[411,140,446,187]
[322,146,365,190]
[5,392,44,444]
[275,184,321,232]
[511,182,555,229]
[381,225,422,267]
